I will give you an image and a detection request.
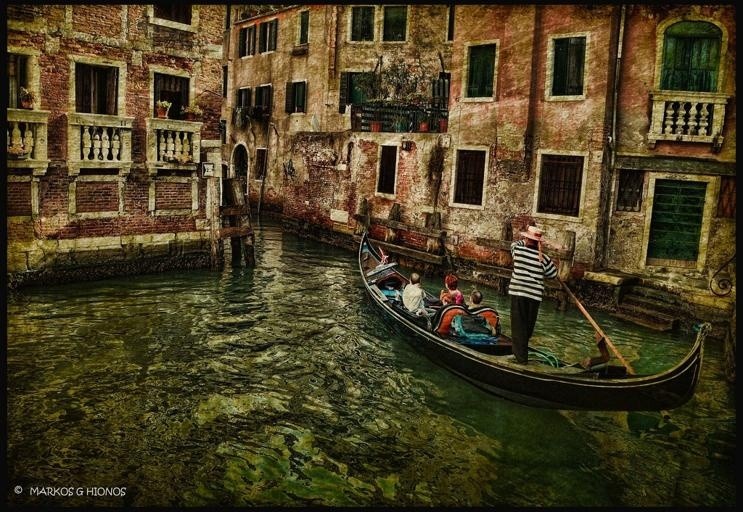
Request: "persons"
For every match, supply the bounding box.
[402,273,437,317]
[508,226,557,365]
[467,290,483,310]
[439,273,464,306]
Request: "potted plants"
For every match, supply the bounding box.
[182,100,202,121]
[20,87,37,109]
[434,114,446,131]
[157,100,172,119]
[369,100,384,131]
[417,113,429,131]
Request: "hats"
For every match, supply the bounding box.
[520,226,546,242]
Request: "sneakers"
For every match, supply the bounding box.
[506,357,528,365]
